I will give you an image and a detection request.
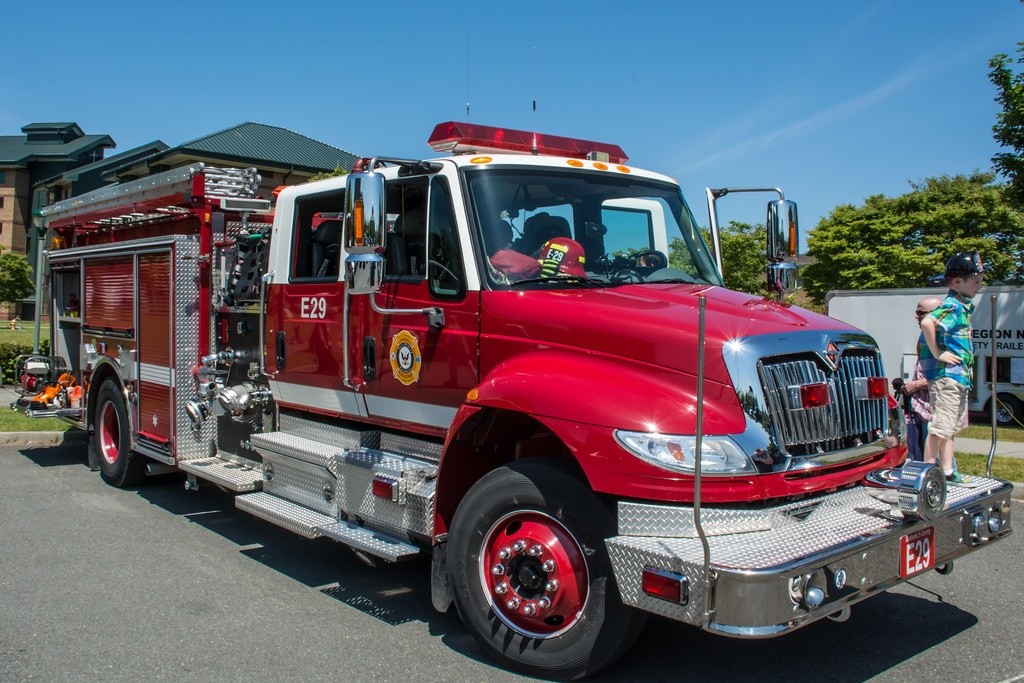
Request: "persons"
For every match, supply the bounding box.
[901,296,943,460]
[920,254,983,487]
[442,220,513,281]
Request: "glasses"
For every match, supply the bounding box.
[915,309,933,315]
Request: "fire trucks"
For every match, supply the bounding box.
[7,118,1018,679]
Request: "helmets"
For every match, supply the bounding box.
[536,236,590,279]
[943,251,987,277]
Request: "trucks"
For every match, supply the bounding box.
[827,287,1024,424]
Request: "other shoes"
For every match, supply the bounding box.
[947,470,980,488]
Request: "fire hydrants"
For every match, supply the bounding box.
[10,319,16,330]
